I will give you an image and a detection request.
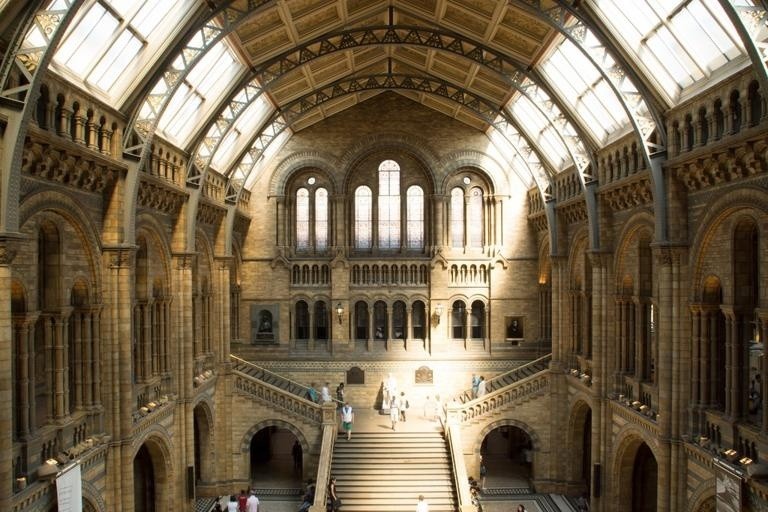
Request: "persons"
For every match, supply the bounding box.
[381,374,396,410]
[340,402,354,441]
[328,476,338,512]
[508,319,522,344]
[321,382,329,404]
[307,382,318,404]
[298,478,316,512]
[389,396,399,431]
[480,456,486,488]
[336,383,344,408]
[416,495,429,512]
[434,395,443,425]
[467,476,483,512]
[472,374,478,399]
[424,396,431,416]
[216,489,259,512]
[476,376,485,398]
[517,504,527,512]
[258,316,271,331]
[399,392,407,422]
[579,492,589,512]
[292,441,300,470]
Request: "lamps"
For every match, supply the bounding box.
[132,394,175,424]
[434,302,444,324]
[333,302,345,326]
[14,434,109,492]
[568,365,755,470]
[194,368,217,387]
